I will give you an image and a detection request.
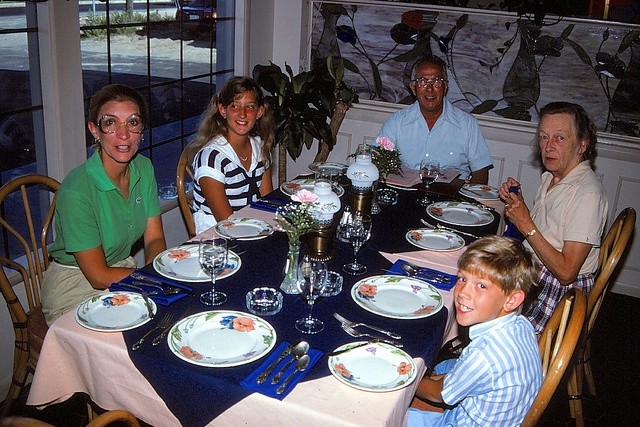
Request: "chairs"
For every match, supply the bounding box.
[4,409,140,426]
[517,287,587,426]
[1,173,84,416]
[570,207,636,426]
[176,135,212,236]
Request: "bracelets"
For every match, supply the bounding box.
[522,226,538,245]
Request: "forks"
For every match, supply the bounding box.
[420,217,482,239]
[333,313,402,339]
[132,312,175,350]
[340,322,404,348]
[256,338,302,384]
[152,304,197,346]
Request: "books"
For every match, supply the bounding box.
[380,160,465,195]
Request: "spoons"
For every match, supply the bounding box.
[271,341,309,385]
[276,355,311,395]
[118,282,159,296]
[132,281,180,295]
[400,262,451,285]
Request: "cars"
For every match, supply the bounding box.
[176,1,217,37]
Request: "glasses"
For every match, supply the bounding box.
[412,77,447,87]
[95,113,145,134]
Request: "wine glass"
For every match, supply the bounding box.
[295,260,327,335]
[199,237,228,306]
[342,213,372,276]
[419,159,440,207]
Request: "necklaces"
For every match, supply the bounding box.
[236,151,253,168]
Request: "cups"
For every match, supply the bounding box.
[313,168,339,190]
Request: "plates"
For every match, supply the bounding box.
[167,309,277,367]
[406,227,466,252]
[426,201,494,226]
[153,244,241,283]
[328,341,414,392]
[458,183,500,200]
[350,274,444,320]
[309,162,350,176]
[75,291,157,333]
[280,179,345,198]
[214,217,274,241]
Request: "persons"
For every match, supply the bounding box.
[497,102,609,341]
[40,84,167,328]
[192,76,274,237]
[406,236,544,427]
[372,57,493,186]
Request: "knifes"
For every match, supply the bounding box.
[141,291,155,318]
[130,273,198,297]
[326,338,379,356]
